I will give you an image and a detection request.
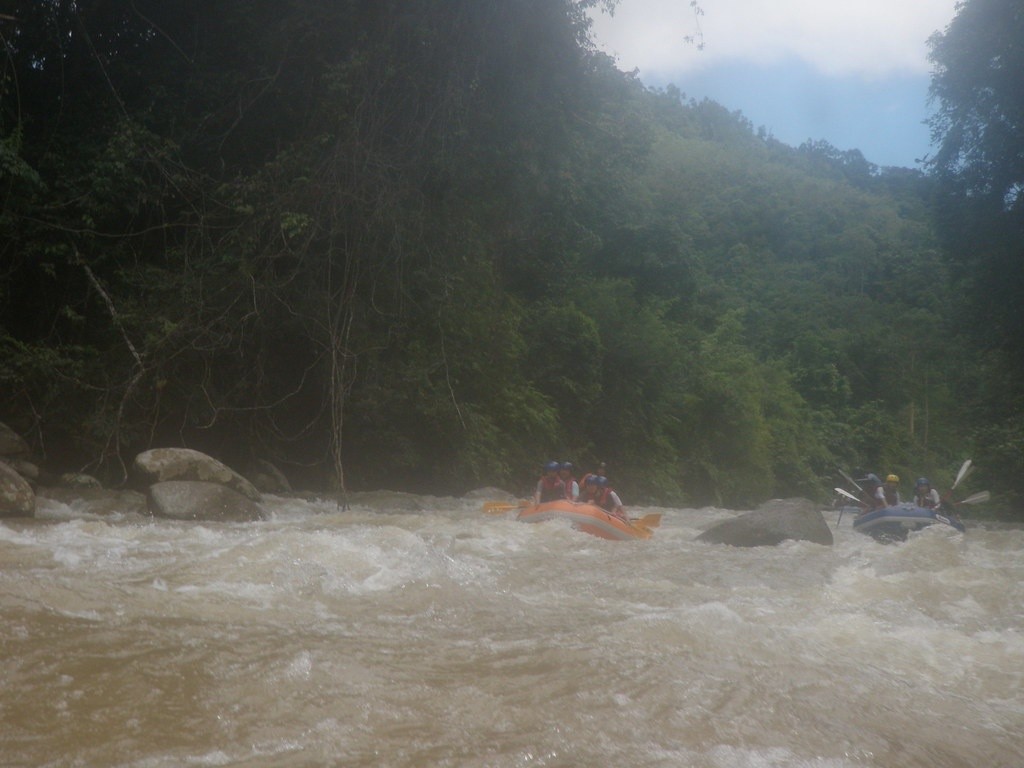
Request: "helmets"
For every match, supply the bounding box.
[546,461,560,472]
[886,474,900,483]
[917,478,930,487]
[597,462,608,472]
[595,476,608,487]
[560,462,573,471]
[584,477,596,485]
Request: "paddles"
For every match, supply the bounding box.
[483,499,662,529]
[834,459,989,527]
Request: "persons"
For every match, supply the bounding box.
[858,470,943,513]
[533,459,630,521]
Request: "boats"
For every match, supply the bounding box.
[853,508,964,544]
[515,499,654,540]
